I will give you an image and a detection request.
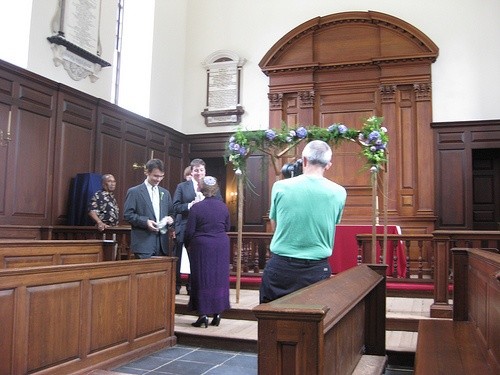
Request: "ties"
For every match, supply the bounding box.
[197,182,201,192]
[152,187,160,222]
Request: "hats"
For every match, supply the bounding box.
[204,175,216,186]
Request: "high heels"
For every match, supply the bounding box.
[191,316,208,328]
[209,314,220,326]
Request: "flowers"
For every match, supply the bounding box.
[160,191,165,200]
[224,117,390,176]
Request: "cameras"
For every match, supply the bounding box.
[281,157,303,179]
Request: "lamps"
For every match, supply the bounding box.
[229,191,238,214]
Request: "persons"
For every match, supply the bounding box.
[173,158,223,294]
[184,176,231,327]
[122,158,175,259]
[259,139,347,302]
[88,174,120,260]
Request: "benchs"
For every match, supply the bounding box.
[416,246,500,375]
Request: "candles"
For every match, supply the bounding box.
[375,195,379,209]
